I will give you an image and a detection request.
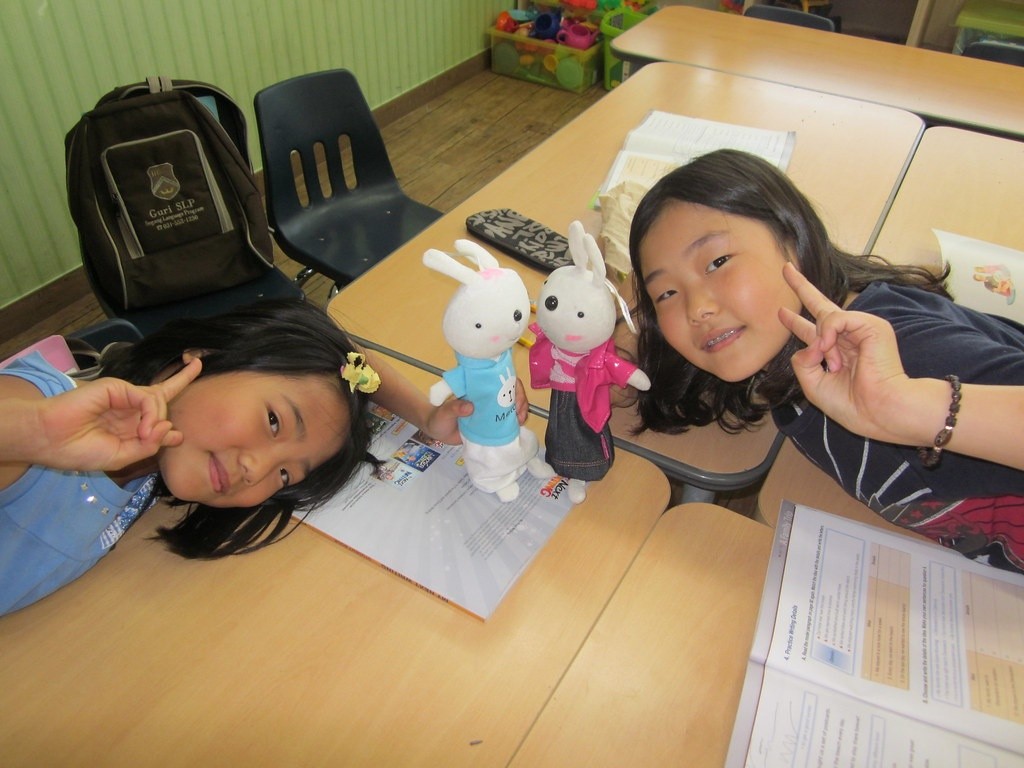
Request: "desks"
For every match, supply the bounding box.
[0,3,1024,768]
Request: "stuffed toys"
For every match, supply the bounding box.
[529,220,651,504]
[423,237,556,502]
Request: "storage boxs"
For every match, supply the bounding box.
[484,22,605,95]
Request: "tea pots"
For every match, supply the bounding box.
[528,8,561,38]
[556,23,600,51]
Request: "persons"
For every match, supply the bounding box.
[610,150,1024,577]
[0,296,529,618]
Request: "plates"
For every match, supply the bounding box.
[507,9,535,24]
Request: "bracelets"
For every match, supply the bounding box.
[917,374,962,468]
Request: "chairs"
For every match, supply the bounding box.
[743,5,834,33]
[252,69,445,300]
[75,221,307,343]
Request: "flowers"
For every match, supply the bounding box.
[340,350,381,394]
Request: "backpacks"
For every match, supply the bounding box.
[63,73,281,316]
